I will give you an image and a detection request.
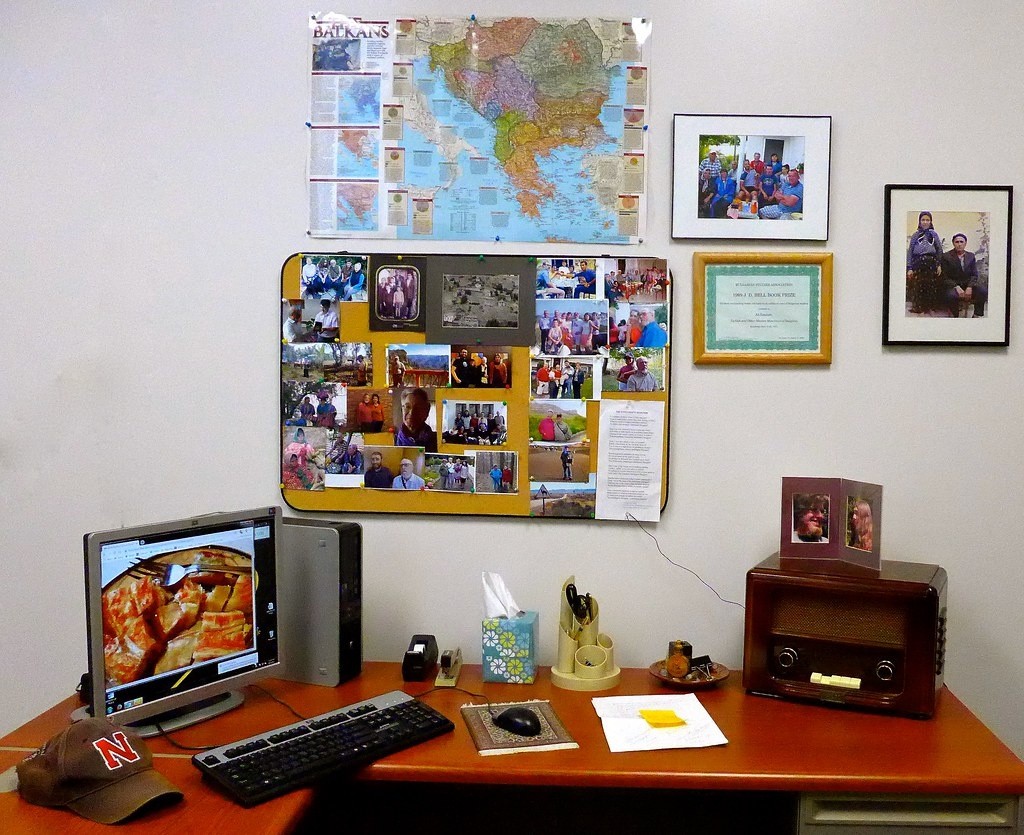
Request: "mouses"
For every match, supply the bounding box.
[492,706,541,737]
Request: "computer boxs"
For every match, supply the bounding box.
[278,516,363,688]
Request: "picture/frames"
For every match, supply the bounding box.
[671,114,832,241]
[880,183,1013,347]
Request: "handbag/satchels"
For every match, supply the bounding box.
[556,422,567,434]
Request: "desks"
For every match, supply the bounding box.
[0,660,1024,835]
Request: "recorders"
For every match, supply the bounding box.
[742,548,949,721]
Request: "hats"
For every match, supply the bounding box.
[624,352,634,358]
[952,233,967,241]
[709,151,716,154]
[565,446,569,448]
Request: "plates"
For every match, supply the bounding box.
[102,545,254,692]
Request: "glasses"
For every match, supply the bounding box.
[399,463,412,468]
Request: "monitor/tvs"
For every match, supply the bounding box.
[82,504,288,728]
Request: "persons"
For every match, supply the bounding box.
[792,492,829,544]
[908,211,946,317]
[943,229,985,319]
[283,246,669,505]
[845,495,874,553]
[700,140,804,224]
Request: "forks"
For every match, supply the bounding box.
[126,556,252,587]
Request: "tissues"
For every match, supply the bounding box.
[478,557,540,686]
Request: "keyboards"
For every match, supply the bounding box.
[191,689,456,810]
